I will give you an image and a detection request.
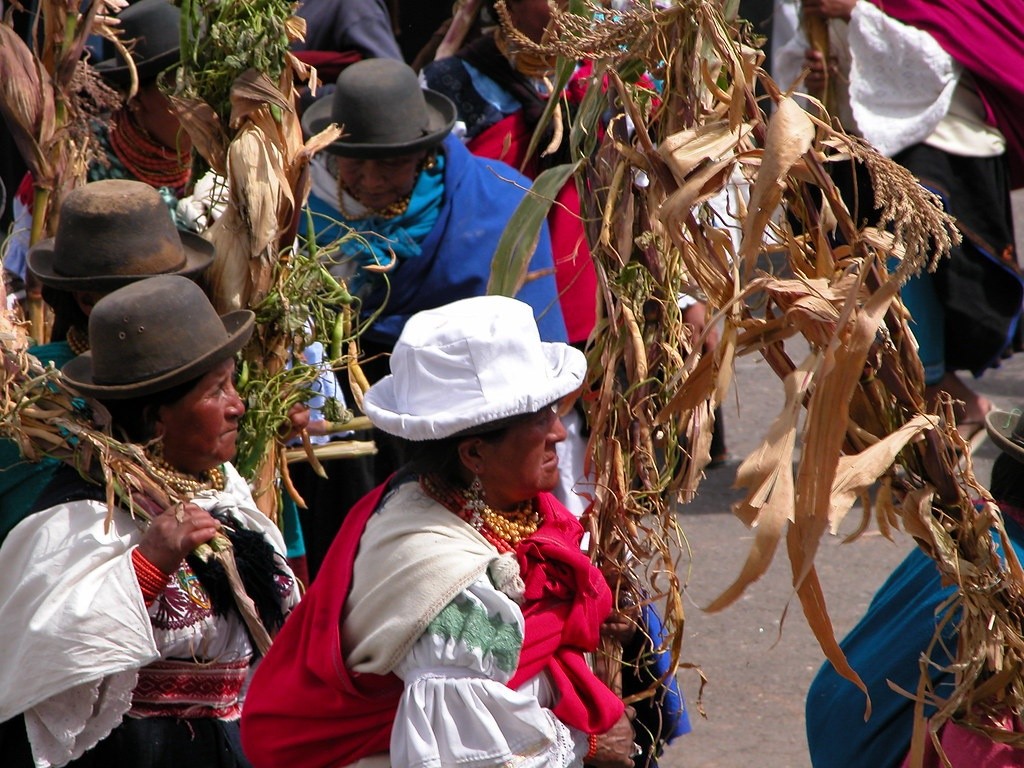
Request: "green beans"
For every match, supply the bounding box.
[539,56,750,559]
[843,419,902,544]
[0,397,74,451]
[234,207,396,510]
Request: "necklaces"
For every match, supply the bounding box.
[419,472,542,555]
[146,453,227,492]
[65,324,90,354]
[326,153,411,221]
[107,109,192,187]
[493,26,557,78]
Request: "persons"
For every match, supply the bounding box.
[342,295,641,768]
[0,0,1024,767]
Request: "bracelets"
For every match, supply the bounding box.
[583,734,597,762]
[125,549,165,608]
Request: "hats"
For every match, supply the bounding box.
[93,0,206,72]
[62,275,255,400]
[984,410,1024,462]
[28,179,216,291]
[300,58,458,157]
[361,295,588,440]
